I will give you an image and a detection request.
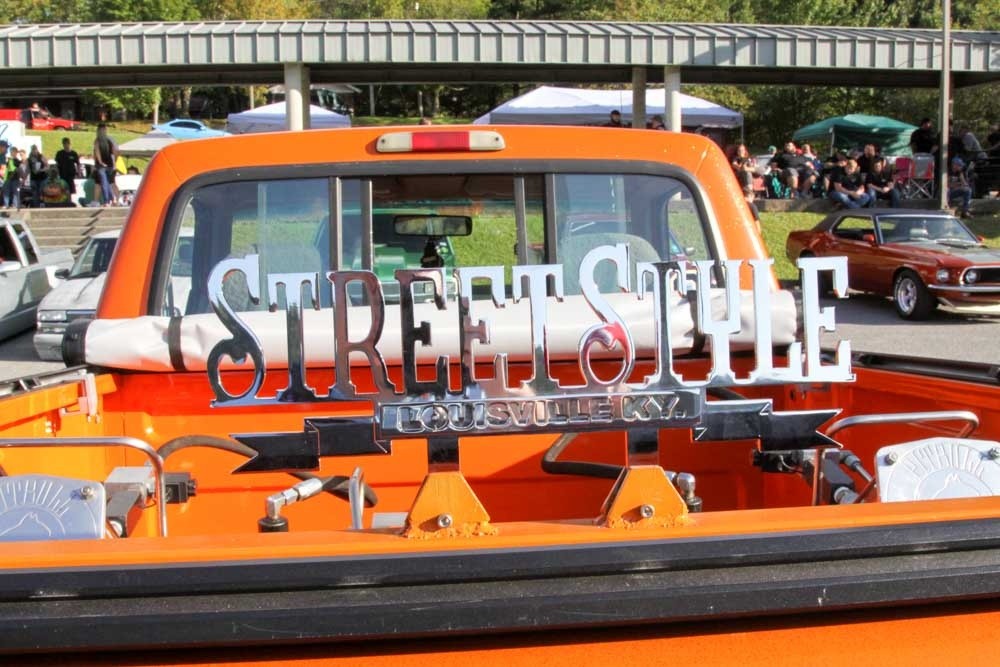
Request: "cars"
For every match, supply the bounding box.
[786,206,1000,322]
[34,226,194,362]
[152,119,233,140]
[0,109,80,132]
[307,208,461,309]
[0,218,75,343]
[513,211,716,298]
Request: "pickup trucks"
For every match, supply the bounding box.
[0,125,998,665]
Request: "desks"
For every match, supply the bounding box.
[68,175,142,207]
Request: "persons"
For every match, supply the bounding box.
[119,190,135,206]
[29,102,48,128]
[730,118,1000,236]
[93,123,119,207]
[419,118,434,124]
[600,110,666,130]
[0,136,80,208]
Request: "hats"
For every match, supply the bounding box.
[650,115,662,123]
[952,157,966,167]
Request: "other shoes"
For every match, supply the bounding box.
[794,191,800,200]
[961,211,975,219]
[112,196,117,205]
[743,186,753,194]
[801,191,809,199]
[85,201,100,206]
[101,204,111,208]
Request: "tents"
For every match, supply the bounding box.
[117,118,233,174]
[473,85,744,143]
[793,114,917,156]
[224,83,362,135]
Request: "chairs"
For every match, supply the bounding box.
[559,232,663,293]
[895,153,935,199]
[770,169,825,197]
[225,245,320,311]
